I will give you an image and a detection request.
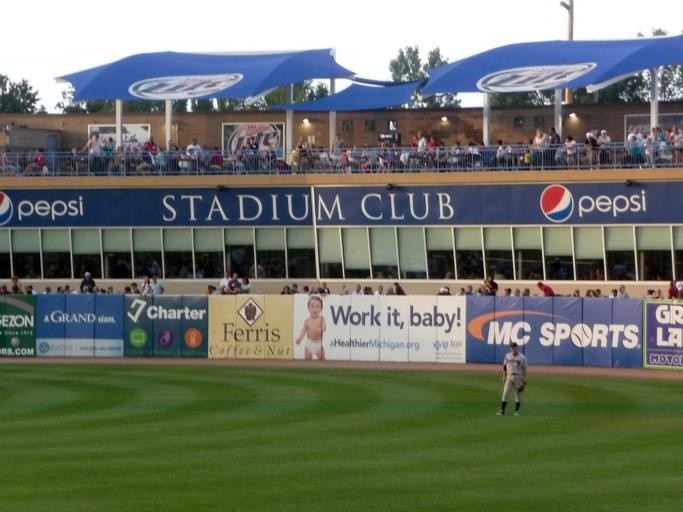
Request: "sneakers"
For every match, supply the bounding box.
[512,410,519,416]
[496,409,503,416]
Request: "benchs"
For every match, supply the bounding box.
[0,147,537,178]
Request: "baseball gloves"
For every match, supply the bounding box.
[515,380,526,392]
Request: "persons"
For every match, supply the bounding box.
[295,297,327,361]
[495,343,526,417]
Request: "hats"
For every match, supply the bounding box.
[510,343,518,348]
